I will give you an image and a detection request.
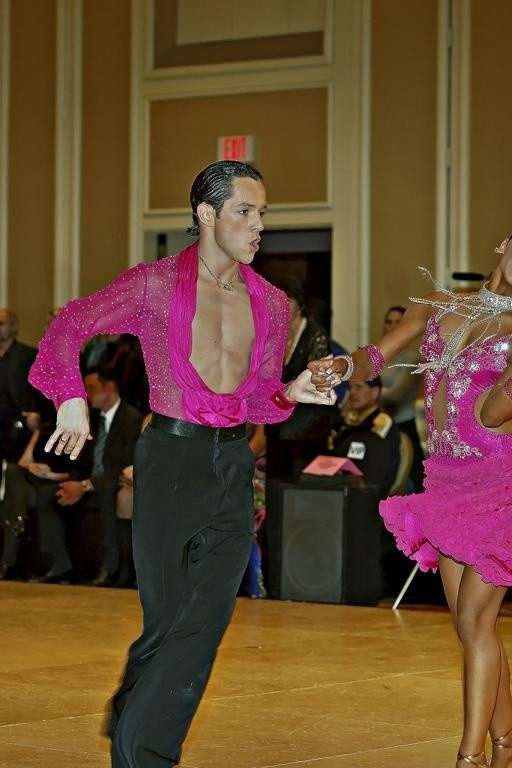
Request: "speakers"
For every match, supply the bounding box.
[279,482,382,604]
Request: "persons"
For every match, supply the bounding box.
[249,261,335,601]
[304,233,512,766]
[0,308,152,590]
[331,375,398,601]
[377,305,425,494]
[26,157,340,766]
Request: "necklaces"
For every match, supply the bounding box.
[198,255,240,293]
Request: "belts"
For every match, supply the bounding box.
[149,411,250,443]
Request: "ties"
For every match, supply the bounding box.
[91,413,108,476]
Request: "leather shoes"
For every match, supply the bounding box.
[26,556,124,587]
